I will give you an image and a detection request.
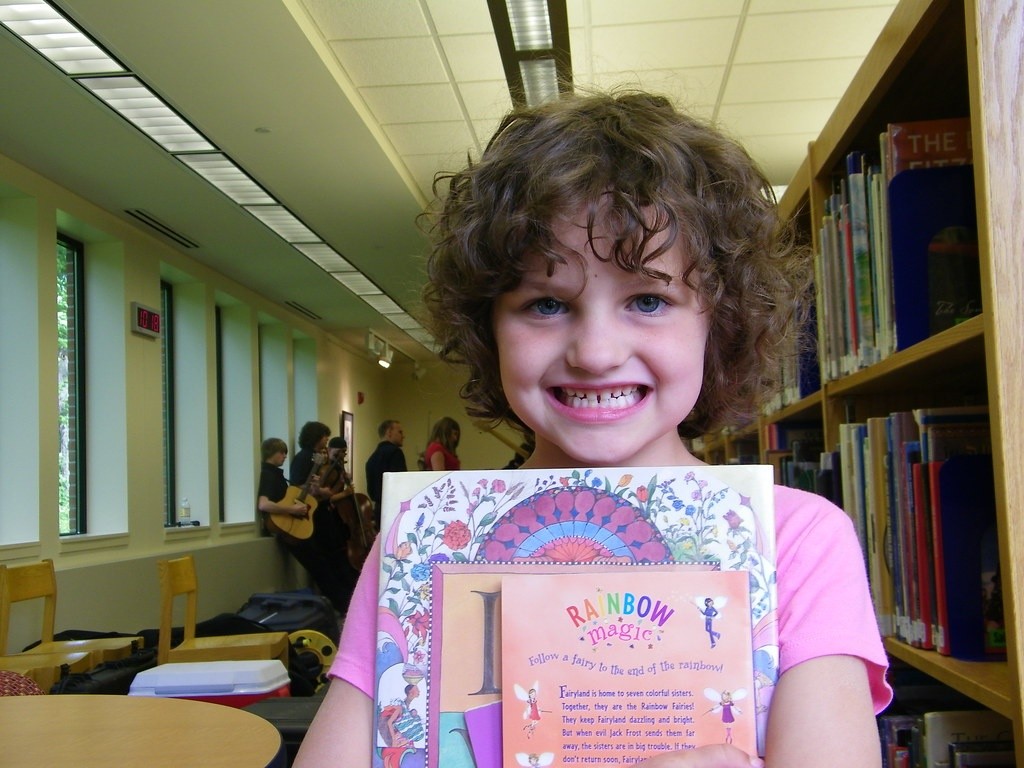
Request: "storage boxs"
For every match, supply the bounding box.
[129,659,290,708]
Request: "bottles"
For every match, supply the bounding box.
[179,498,190,525]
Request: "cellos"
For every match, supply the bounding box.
[333,450,377,570]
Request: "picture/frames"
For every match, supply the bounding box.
[341,410,353,481]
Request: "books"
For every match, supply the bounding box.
[734,120,1018,768]
[372,464,778,768]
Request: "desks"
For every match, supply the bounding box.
[0,695,283,768]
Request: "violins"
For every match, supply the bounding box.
[307,454,340,504]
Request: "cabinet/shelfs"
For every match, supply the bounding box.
[690,0,1024,768]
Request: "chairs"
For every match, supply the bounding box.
[0,651,88,694]
[0,559,144,669]
[156,554,289,670]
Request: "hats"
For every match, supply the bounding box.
[328,437,347,448]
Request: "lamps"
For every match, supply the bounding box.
[378,343,393,368]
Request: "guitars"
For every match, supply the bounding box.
[264,452,327,546]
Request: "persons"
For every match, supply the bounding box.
[366,420,408,531]
[424,416,462,471]
[259,422,355,612]
[293,92,894,768]
